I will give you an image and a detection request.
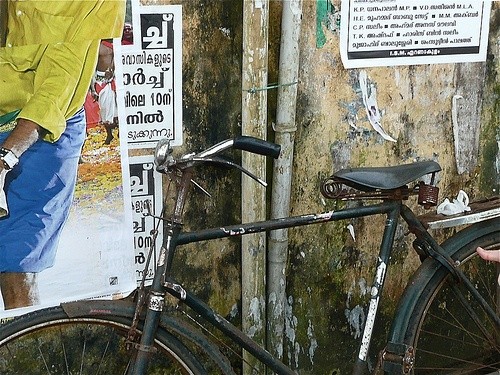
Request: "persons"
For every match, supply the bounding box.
[476,247,500,286]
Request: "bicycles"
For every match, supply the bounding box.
[0,134,500,375]
